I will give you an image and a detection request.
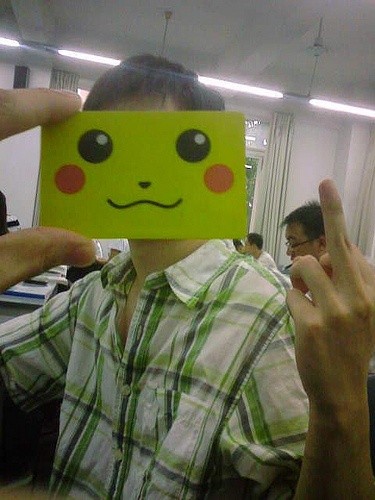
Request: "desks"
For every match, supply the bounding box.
[0,272,66,324]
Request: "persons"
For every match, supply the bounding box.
[279,198,375,477]
[0,54,375,500]
[233,239,245,254]
[245,233,278,271]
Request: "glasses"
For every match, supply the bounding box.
[285,236,320,251]
